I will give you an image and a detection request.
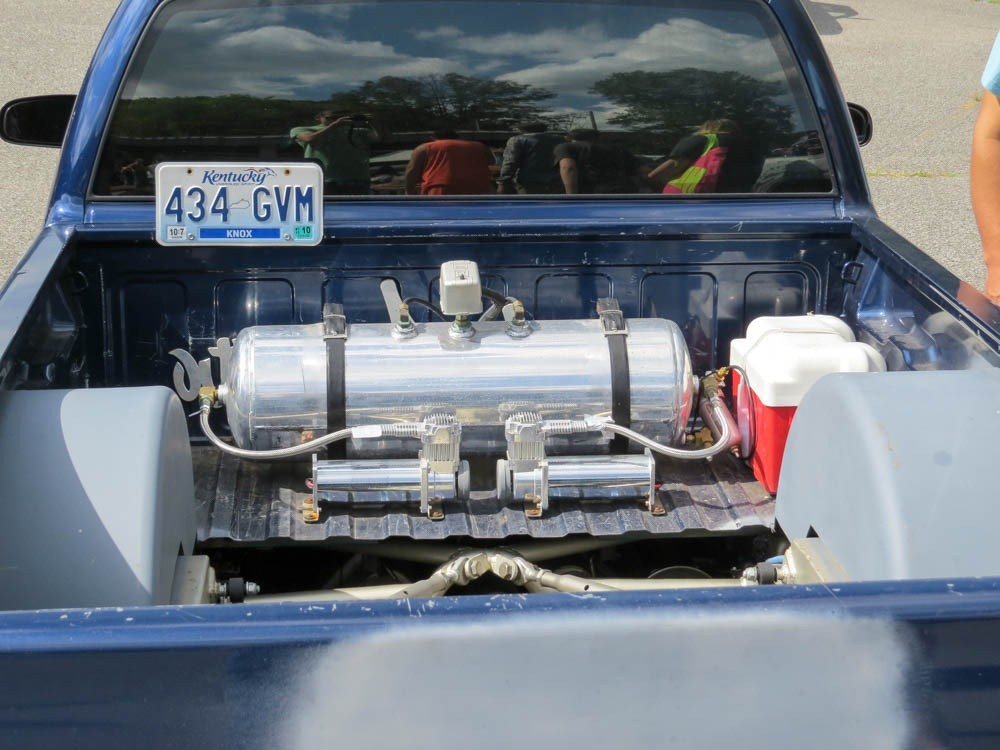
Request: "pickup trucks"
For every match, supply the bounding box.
[0,2,998,750]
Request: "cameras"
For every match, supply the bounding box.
[349,113,367,121]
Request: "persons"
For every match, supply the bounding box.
[554,129,640,194]
[495,122,565,194]
[971,28,1000,302]
[405,129,495,195]
[647,119,766,193]
[290,106,380,195]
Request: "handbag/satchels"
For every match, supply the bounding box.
[662,130,728,195]
